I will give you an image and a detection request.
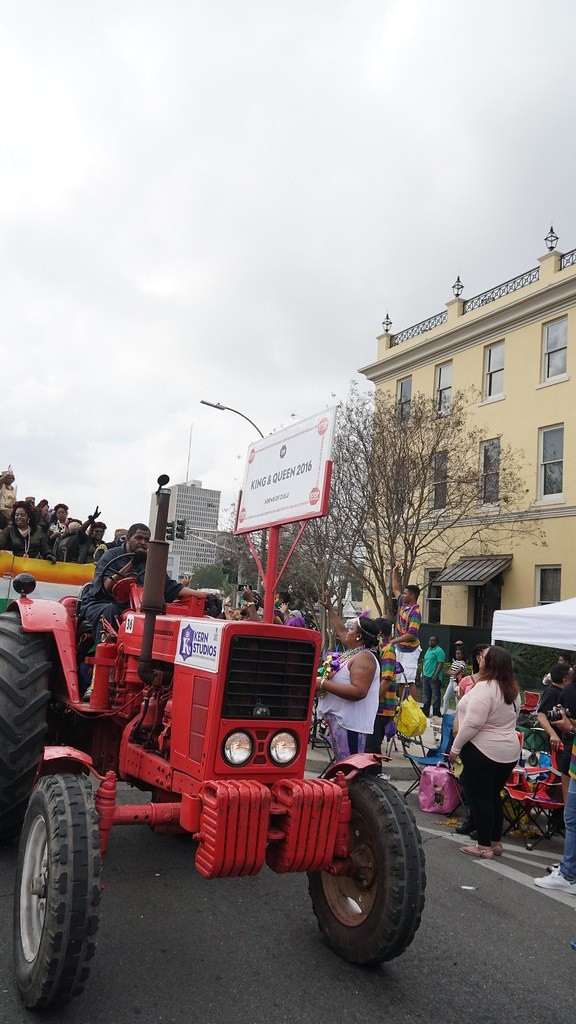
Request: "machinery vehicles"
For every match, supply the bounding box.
[0,474,425,1014]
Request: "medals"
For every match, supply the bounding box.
[23,554,29,558]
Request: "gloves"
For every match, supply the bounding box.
[7,464,12,475]
[131,548,148,567]
[47,554,56,565]
[88,506,101,523]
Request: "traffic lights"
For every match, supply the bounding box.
[176,518,185,539]
[166,520,175,540]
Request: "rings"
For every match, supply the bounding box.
[551,743,553,744]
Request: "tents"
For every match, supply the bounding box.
[491,595,576,653]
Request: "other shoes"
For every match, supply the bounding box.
[456,825,469,835]
[469,831,479,840]
[82,686,92,702]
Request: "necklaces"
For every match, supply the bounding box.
[318,645,366,680]
[400,605,418,635]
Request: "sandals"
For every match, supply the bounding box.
[490,844,503,856]
[460,844,493,858]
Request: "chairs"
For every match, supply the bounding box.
[519,690,540,715]
[59,596,80,630]
[403,714,471,820]
[317,733,336,779]
[500,752,566,851]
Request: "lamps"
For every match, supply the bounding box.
[544,226,560,252]
[382,314,393,333]
[452,276,464,297]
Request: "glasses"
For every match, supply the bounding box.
[93,529,104,534]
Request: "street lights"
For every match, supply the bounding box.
[200,399,268,593]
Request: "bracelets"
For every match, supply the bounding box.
[320,679,325,691]
[569,726,576,735]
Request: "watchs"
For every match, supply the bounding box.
[247,602,255,606]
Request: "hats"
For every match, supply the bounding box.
[289,610,301,617]
[2,470,13,475]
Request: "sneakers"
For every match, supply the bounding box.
[534,867,576,894]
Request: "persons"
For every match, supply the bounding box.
[77,507,107,565]
[315,590,380,761]
[0,464,82,565]
[389,558,421,699]
[442,649,465,714]
[450,644,521,858]
[366,618,396,756]
[180,575,318,630]
[445,641,467,663]
[533,654,576,895]
[421,635,446,717]
[93,529,127,561]
[81,524,213,646]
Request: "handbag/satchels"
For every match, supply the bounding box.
[419,761,460,813]
[395,693,427,736]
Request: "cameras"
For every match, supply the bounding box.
[547,708,572,722]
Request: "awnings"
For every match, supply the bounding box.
[432,556,510,586]
